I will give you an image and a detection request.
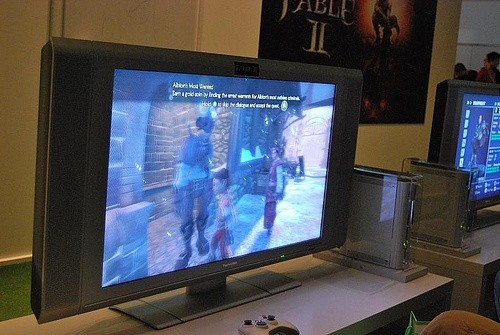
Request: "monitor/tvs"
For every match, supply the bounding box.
[427,79,500,233]
[29,36,365,329]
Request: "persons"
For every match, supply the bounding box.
[454,52,500,84]
[372,0,392,40]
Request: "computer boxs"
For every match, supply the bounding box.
[328,163,422,271]
[405,158,474,246]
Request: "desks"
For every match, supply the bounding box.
[0,251,454,335]
[410,204,500,317]
